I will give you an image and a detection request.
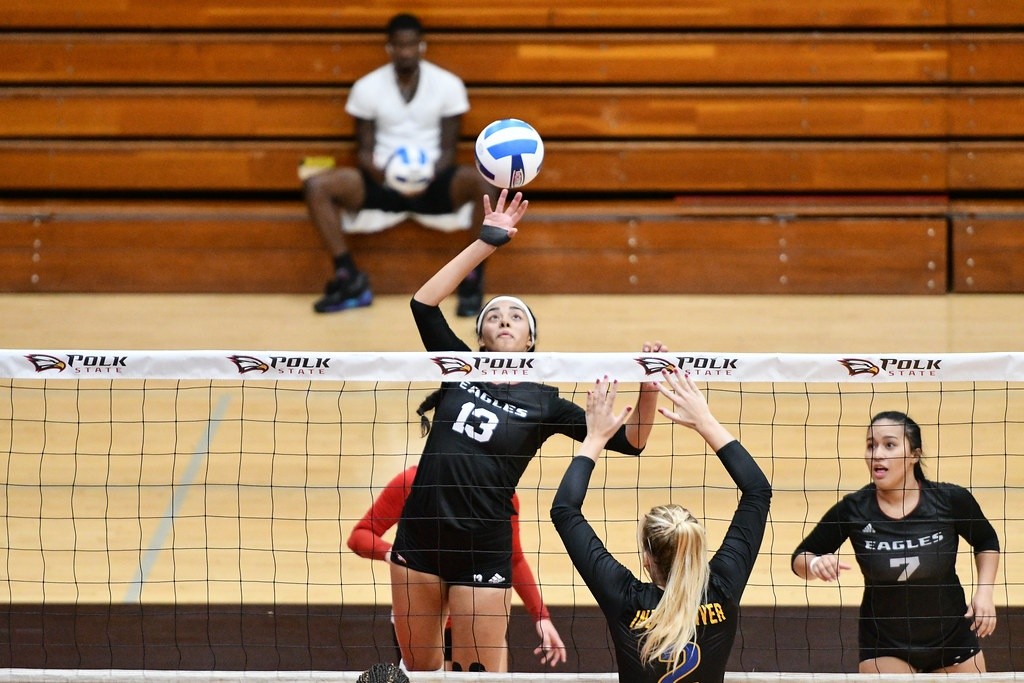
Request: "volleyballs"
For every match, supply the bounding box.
[384,144,433,193]
[475,118,546,188]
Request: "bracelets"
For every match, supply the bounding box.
[809,556,820,576]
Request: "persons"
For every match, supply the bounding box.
[551,365,772,683]
[347,190,670,683]
[791,412,999,674]
[305,13,498,317]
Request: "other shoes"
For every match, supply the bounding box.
[313,272,373,312]
[457,278,483,316]
